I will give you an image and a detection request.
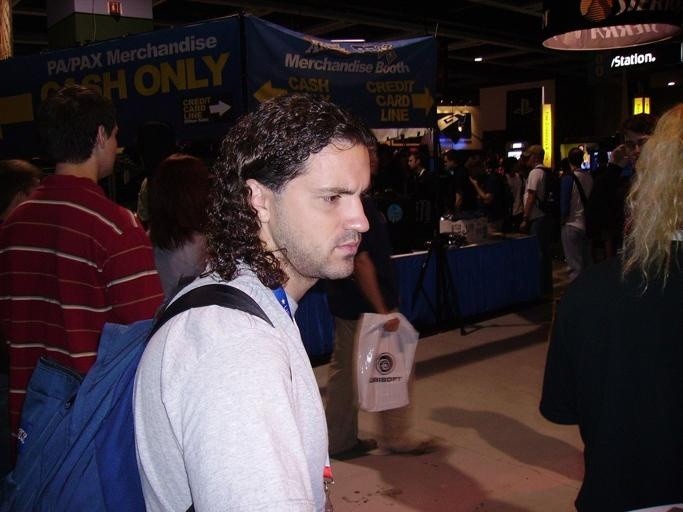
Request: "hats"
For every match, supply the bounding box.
[522,145,543,157]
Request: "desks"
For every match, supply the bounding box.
[296,233,540,366]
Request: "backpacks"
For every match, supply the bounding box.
[534,167,561,216]
[0,276,275,512]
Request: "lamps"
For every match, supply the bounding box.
[540,0,683,52]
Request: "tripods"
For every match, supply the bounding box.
[409,127,468,336]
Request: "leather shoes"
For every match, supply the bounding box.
[329,439,378,459]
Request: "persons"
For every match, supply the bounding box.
[618,113,662,257]
[131,88,371,511]
[534,101,681,512]
[0,82,166,472]
[375,143,482,256]
[323,127,436,459]
[482,140,630,287]
[0,117,213,303]
